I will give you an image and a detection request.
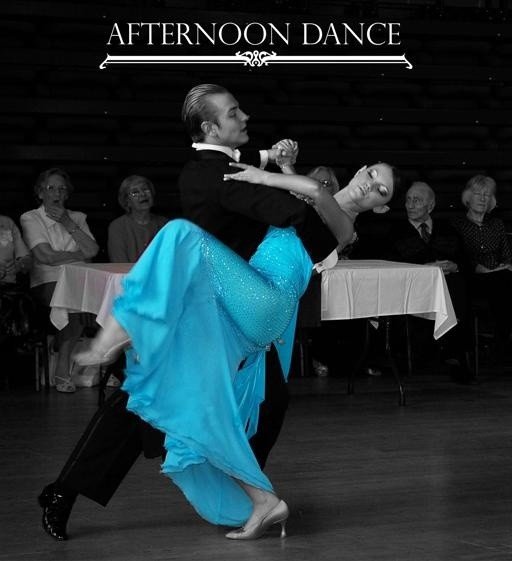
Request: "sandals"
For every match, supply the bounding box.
[53,376,76,393]
[102,370,121,388]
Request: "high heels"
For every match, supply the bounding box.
[73,335,140,368]
[224,500,290,540]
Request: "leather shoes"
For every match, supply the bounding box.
[38,482,76,540]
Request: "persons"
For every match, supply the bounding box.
[308,161,340,198]
[19,167,123,394]
[401,182,481,385]
[36,81,341,541]
[0,208,33,384]
[107,174,170,264]
[72,139,405,541]
[455,175,512,360]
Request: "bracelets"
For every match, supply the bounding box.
[67,228,81,233]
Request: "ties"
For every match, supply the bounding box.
[420,223,430,243]
